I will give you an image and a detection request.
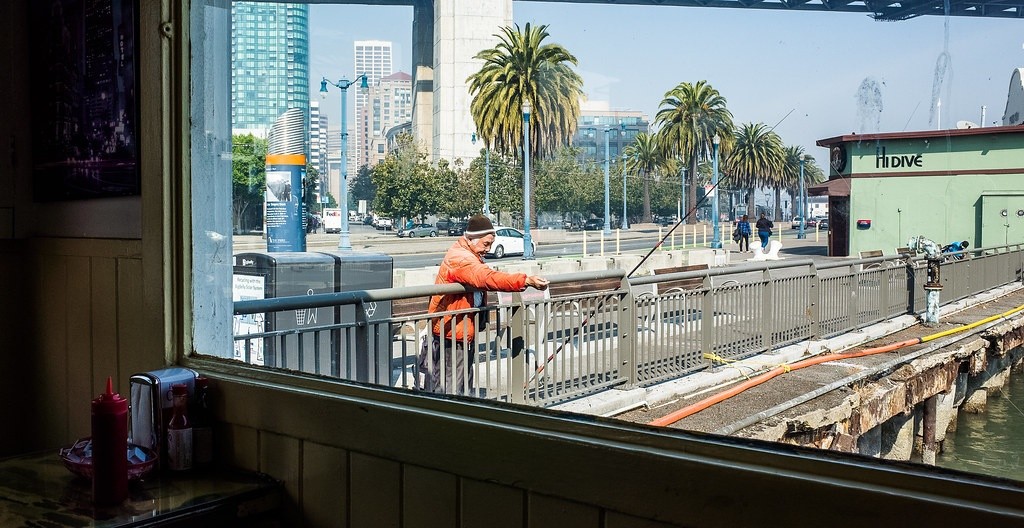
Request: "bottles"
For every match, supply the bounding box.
[192,377,216,479]
[167,384,193,481]
[92,376,128,507]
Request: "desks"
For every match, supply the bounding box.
[0,443,283,528]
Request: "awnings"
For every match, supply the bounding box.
[807,178,851,198]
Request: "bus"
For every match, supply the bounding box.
[730,203,749,226]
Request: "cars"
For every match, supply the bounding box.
[479,226,537,259]
[447,220,468,236]
[348,211,392,231]
[539,216,631,231]
[397,223,439,238]
[435,218,454,230]
[791,215,829,231]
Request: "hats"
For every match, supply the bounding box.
[465,214,495,236]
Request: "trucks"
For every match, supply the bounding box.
[324,208,341,234]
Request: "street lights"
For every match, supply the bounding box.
[470,131,490,219]
[587,121,627,235]
[318,71,370,251]
[611,151,639,231]
[798,151,806,239]
[711,132,723,249]
[520,97,536,261]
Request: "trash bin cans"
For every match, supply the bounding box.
[315,251,393,387]
[232,252,335,378]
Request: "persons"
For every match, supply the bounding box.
[943,240,969,257]
[756,213,774,248]
[425,211,550,395]
[737,214,751,253]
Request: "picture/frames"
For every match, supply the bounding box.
[23,0,141,203]
